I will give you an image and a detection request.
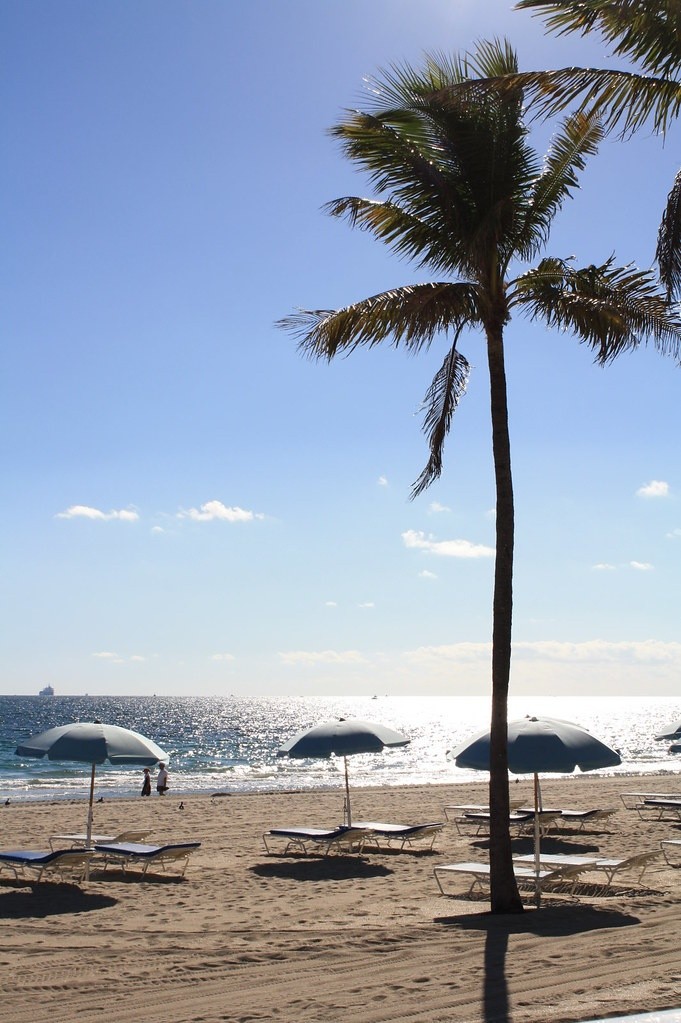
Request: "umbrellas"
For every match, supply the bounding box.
[653,721,681,753]
[452,714,622,891]
[276,718,411,853]
[14,720,170,880]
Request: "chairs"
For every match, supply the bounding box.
[94,843,202,881]
[48,829,154,866]
[517,808,620,833]
[432,861,599,906]
[660,840,681,866]
[444,799,528,824]
[619,793,681,809]
[513,849,667,896]
[635,800,681,822]
[262,826,376,862]
[334,821,447,853]
[454,810,563,840]
[0,847,96,884]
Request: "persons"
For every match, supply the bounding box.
[141,768,151,796]
[157,763,168,795]
